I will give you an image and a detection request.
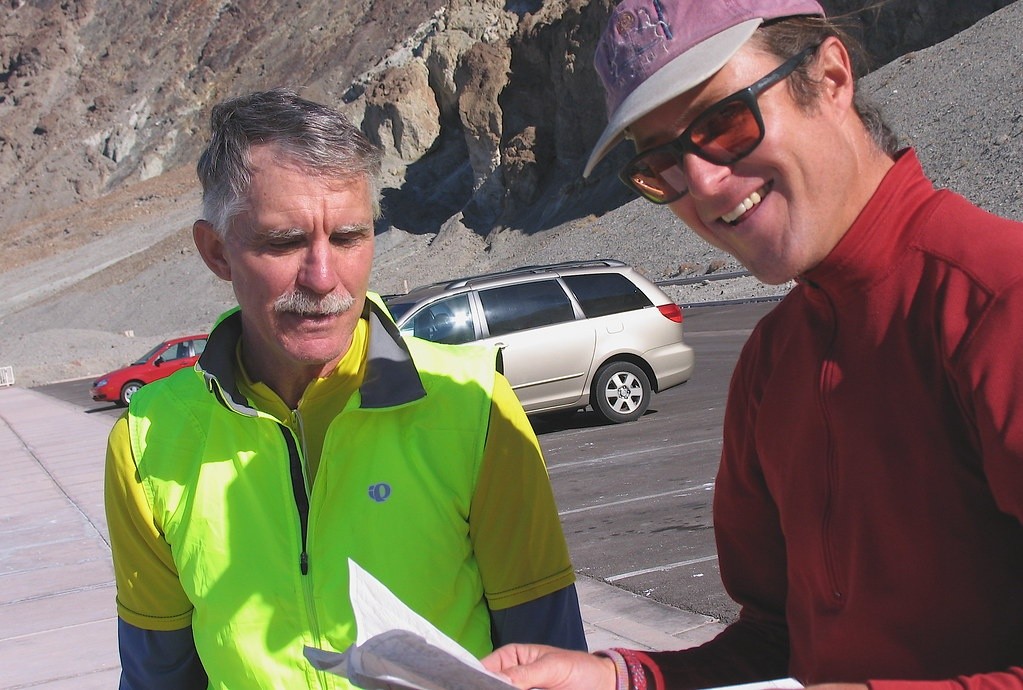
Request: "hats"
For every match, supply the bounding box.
[581,0,827,178]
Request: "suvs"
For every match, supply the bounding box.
[383,258,692,425]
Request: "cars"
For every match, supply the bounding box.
[90,332,213,405]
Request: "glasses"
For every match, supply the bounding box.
[617,45,817,205]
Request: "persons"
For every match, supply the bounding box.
[480,0,1023,690]
[104,87,590,690]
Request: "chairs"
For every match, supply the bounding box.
[178,346,187,357]
[418,312,453,342]
